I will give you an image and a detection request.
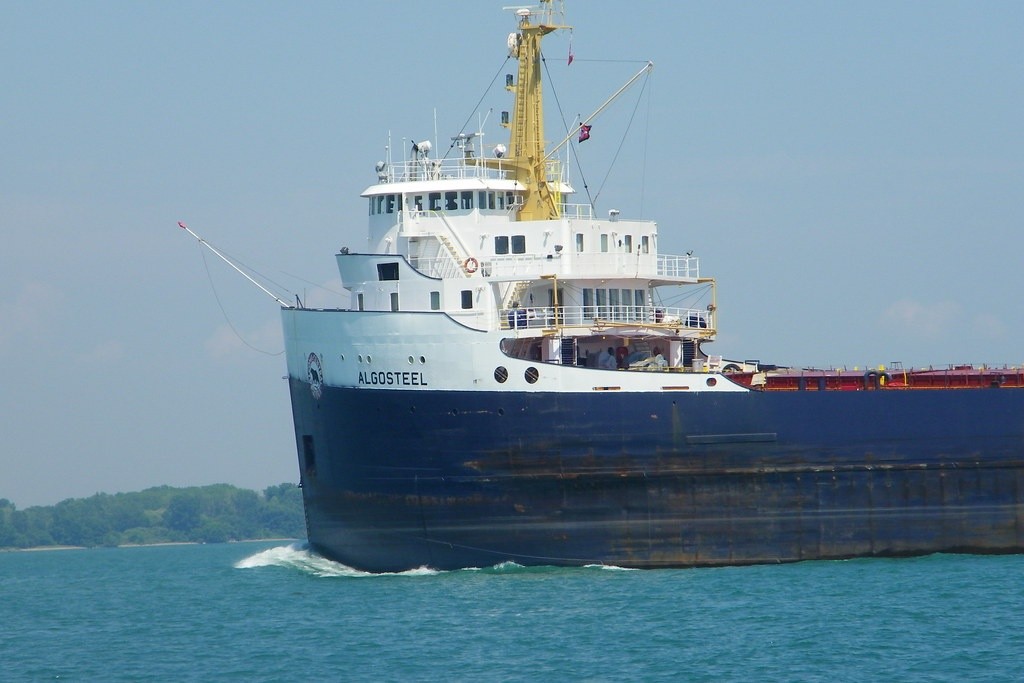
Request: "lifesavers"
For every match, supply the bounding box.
[464,258,478,273]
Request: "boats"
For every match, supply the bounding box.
[281,132,1024,574]
